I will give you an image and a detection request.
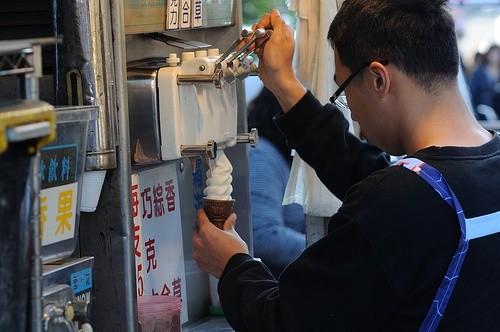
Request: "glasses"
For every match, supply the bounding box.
[330,59,390,108]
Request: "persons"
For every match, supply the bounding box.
[240,76,310,275]
[191,0,500,332]
[454,40,500,125]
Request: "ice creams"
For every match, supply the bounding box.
[203,149,235,230]
